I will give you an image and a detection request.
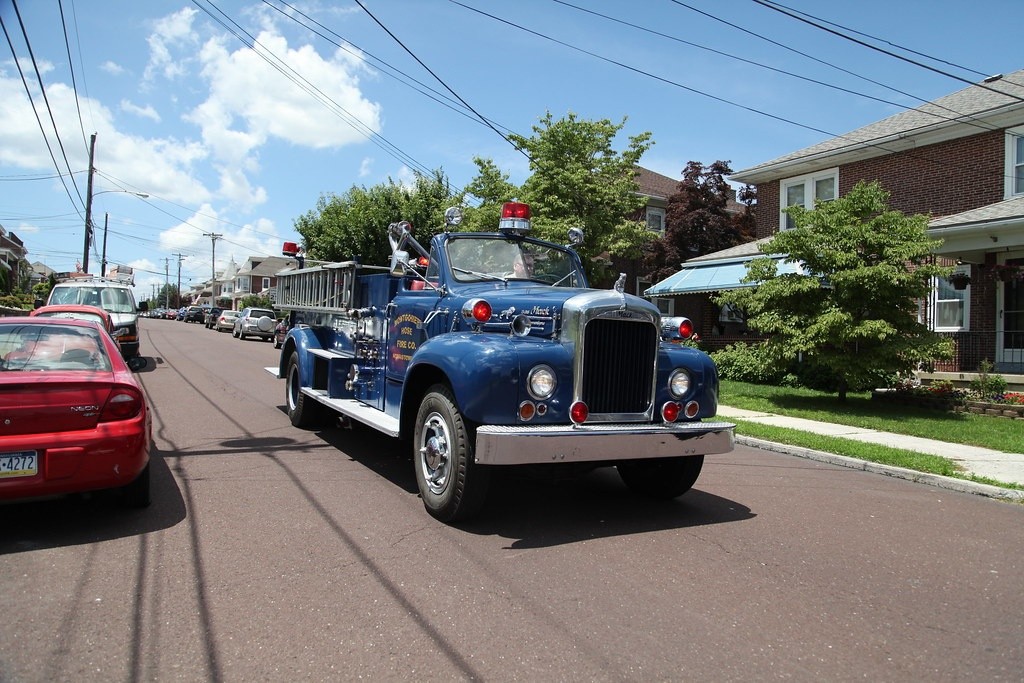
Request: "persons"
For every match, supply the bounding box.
[504,251,536,279]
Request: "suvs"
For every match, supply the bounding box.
[265,200,739,526]
[45,265,141,367]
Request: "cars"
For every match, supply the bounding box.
[141,302,290,348]
[0,316,155,510]
[28,303,129,355]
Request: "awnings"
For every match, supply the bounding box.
[642,255,838,299]
